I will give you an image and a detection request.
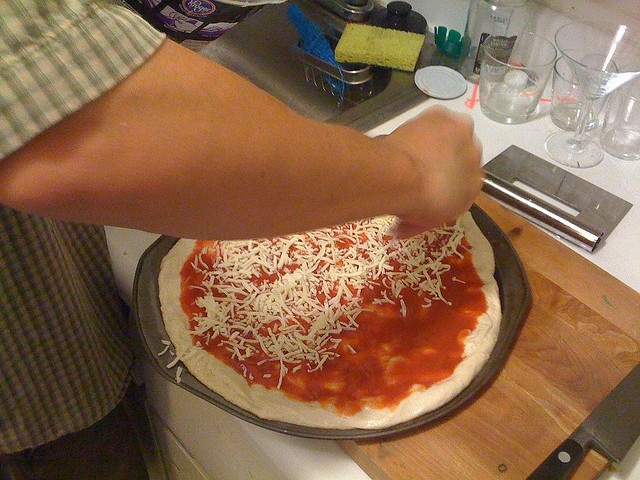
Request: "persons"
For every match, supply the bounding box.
[0,1,485,480]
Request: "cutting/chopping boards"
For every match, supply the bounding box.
[337,193,640,478]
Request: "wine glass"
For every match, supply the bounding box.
[548,23,640,168]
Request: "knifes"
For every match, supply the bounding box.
[512,362,640,479]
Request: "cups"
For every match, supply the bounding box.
[480,29,556,126]
[600,91,639,162]
[454,0,520,82]
[550,57,606,135]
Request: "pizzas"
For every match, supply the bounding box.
[158,211,504,430]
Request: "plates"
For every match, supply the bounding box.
[132,200,530,440]
[415,65,467,100]
